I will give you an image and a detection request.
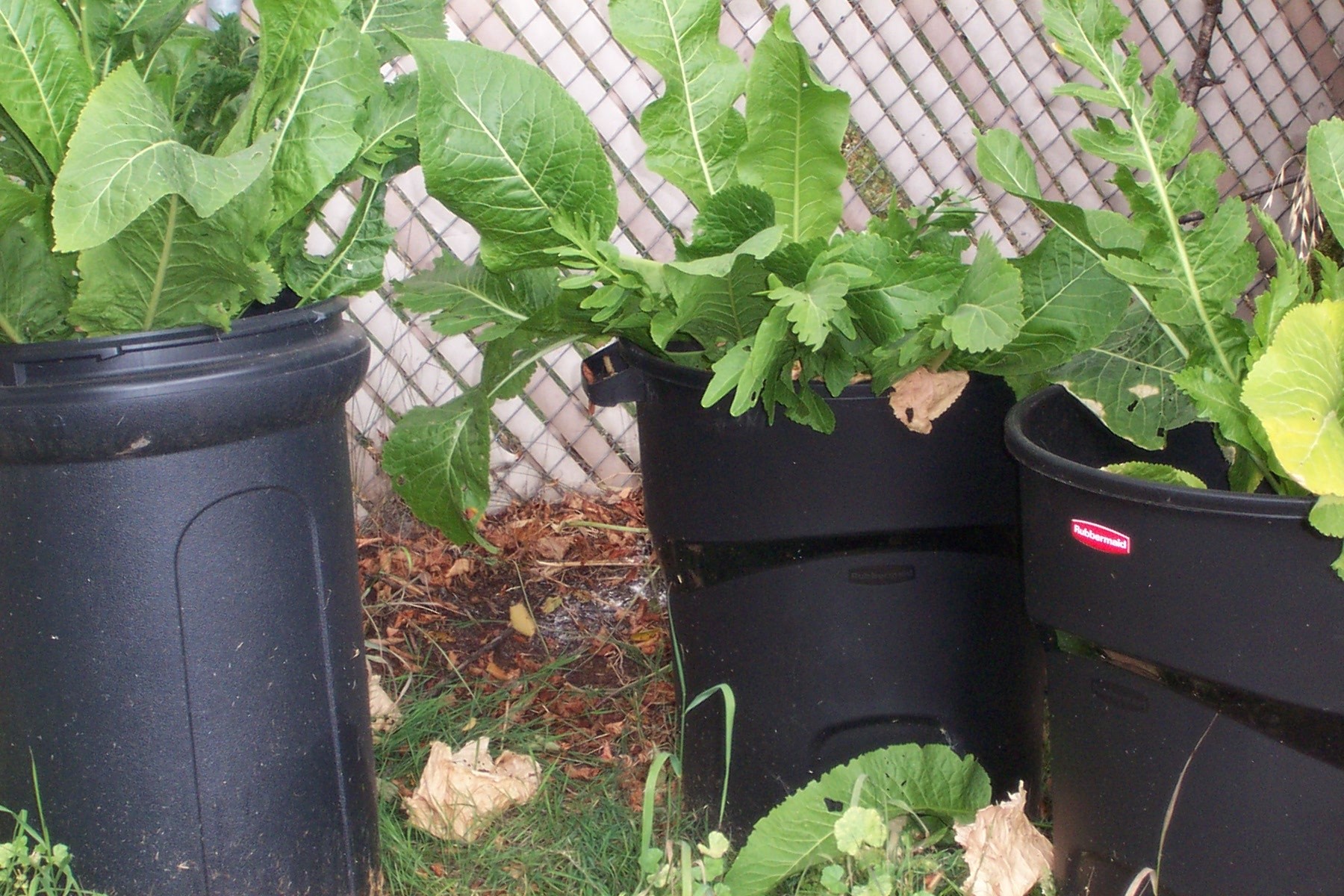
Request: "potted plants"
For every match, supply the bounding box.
[2,0,1343,896]
